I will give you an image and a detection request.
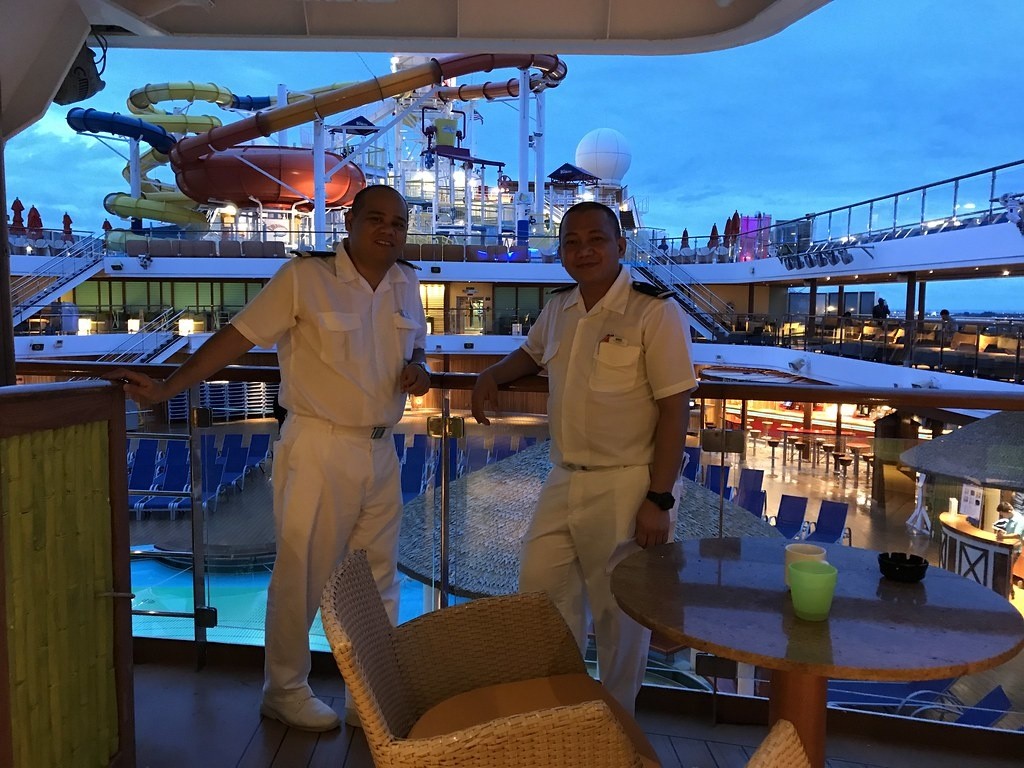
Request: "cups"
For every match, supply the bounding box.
[789,559,837,622]
[785,542,831,589]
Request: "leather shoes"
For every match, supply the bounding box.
[261,686,341,732]
[344,683,362,728]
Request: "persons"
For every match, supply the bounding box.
[997,502,1024,566]
[100,183,430,732]
[873,298,890,332]
[841,312,854,328]
[941,310,958,345]
[471,202,698,719]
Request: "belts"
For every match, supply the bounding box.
[559,464,634,472]
[296,415,394,439]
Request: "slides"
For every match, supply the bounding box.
[66,53,570,239]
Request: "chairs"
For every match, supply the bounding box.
[126,237,529,263]
[804,499,852,547]
[704,465,739,502]
[682,444,705,489]
[124,432,553,522]
[737,468,768,523]
[321,547,814,768]
[767,493,810,542]
[728,315,1024,386]
[846,443,871,476]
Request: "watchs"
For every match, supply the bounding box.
[646,491,676,511]
[411,361,432,377]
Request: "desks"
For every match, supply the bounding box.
[610,536,1024,768]
[777,428,856,466]
[719,413,742,431]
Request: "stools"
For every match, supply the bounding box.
[705,419,874,479]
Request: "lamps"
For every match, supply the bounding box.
[788,354,811,372]
[911,374,943,389]
[778,245,875,271]
